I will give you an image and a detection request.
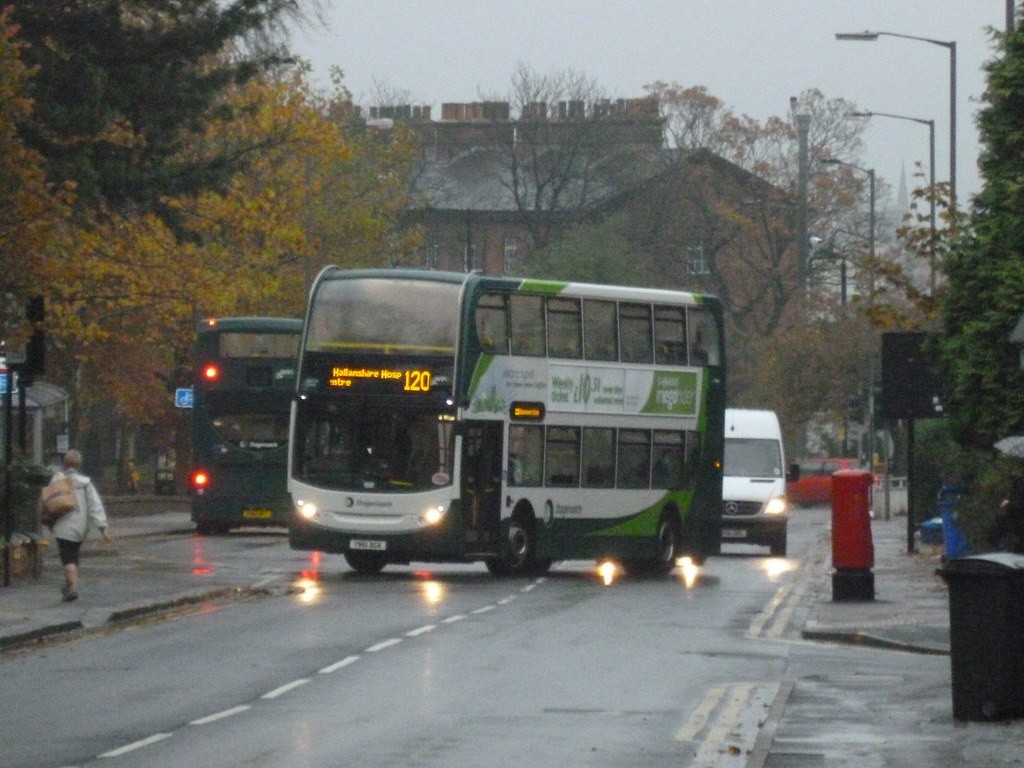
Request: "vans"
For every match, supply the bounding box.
[709,408,800,557]
[784,456,862,505]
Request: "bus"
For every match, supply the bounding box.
[278,261,728,581]
[188,315,308,536]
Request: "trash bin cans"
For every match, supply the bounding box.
[935,550,1024,721]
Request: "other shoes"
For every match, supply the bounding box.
[60,585,78,601]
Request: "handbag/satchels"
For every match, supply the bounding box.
[42,473,75,516]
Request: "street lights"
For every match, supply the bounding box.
[835,30,959,241]
[845,110,937,297]
[820,157,875,510]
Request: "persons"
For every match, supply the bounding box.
[49,449,112,603]
